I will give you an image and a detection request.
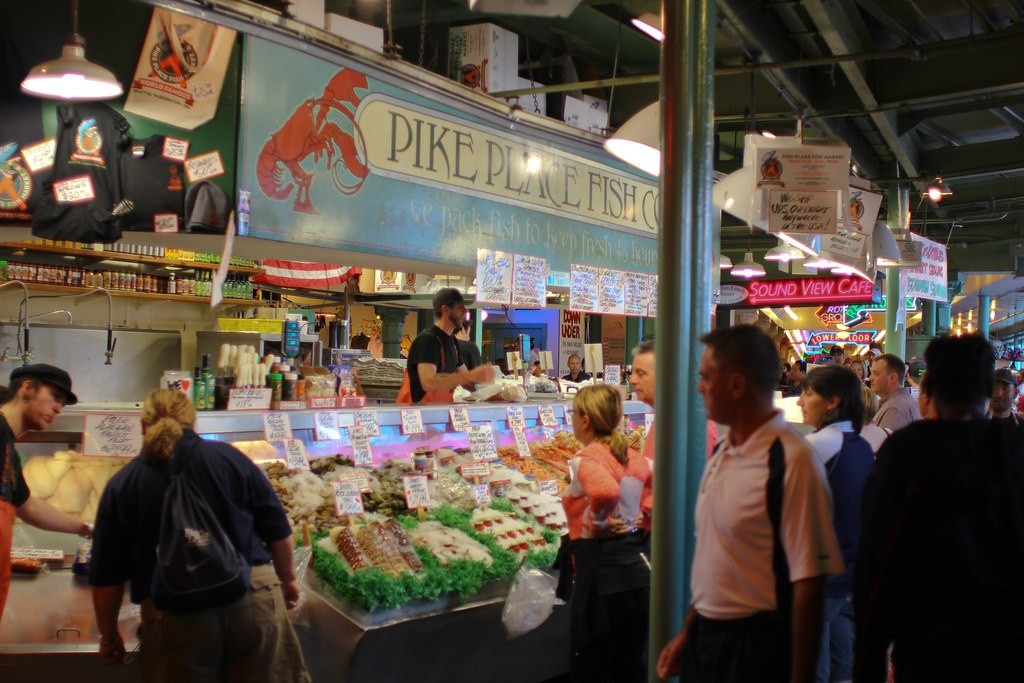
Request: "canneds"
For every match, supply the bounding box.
[6,261,196,296]
[257,355,305,401]
[104,242,166,256]
[414,447,568,554]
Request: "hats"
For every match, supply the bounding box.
[867,348,881,356]
[991,369,1012,385]
[185,180,227,233]
[910,362,926,377]
[9,364,77,406]
[830,345,844,355]
[431,287,477,311]
[454,320,472,327]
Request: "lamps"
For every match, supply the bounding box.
[604,0,902,285]
[20,0,125,103]
[921,176,954,202]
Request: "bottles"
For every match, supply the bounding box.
[192,367,206,409]
[329,321,338,348]
[0,259,254,299]
[215,377,233,409]
[159,370,193,403]
[201,353,215,409]
[266,355,305,402]
[339,325,347,349]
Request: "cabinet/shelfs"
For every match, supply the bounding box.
[0,241,267,307]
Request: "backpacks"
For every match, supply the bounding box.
[32,102,129,244]
[131,439,249,617]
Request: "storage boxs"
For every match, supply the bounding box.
[374,270,477,296]
[216,377,367,410]
[283,0,611,135]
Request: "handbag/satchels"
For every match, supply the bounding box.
[117,134,183,231]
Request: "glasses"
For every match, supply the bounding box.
[832,351,842,354]
[822,357,832,362]
[567,409,583,417]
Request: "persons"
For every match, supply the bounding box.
[0,364,94,631]
[561,354,592,382]
[92,387,313,683]
[453,319,483,391]
[533,361,545,377]
[395,286,495,403]
[798,333,1024,683]
[552,324,848,683]
